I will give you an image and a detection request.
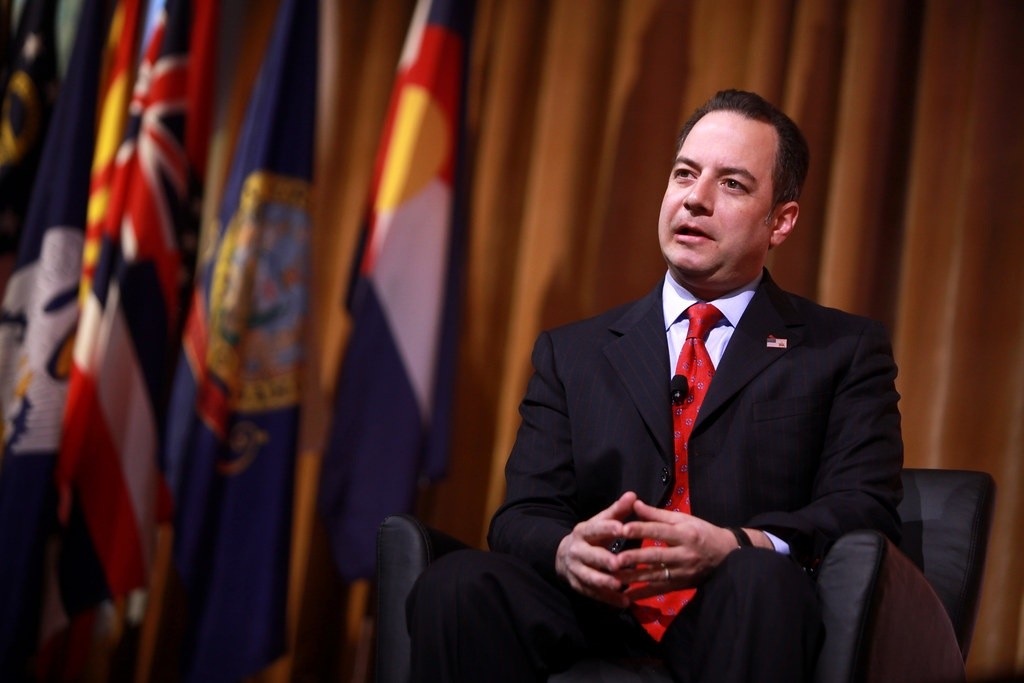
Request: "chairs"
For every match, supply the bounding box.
[376,467,998,683]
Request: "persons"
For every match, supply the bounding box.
[485,89,904,683]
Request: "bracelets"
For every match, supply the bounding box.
[726,516,754,548]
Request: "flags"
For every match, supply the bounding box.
[150,2,318,683]
[58,0,194,629]
[0,0,116,682]
[316,0,472,587]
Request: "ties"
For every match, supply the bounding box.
[627,303,723,643]
